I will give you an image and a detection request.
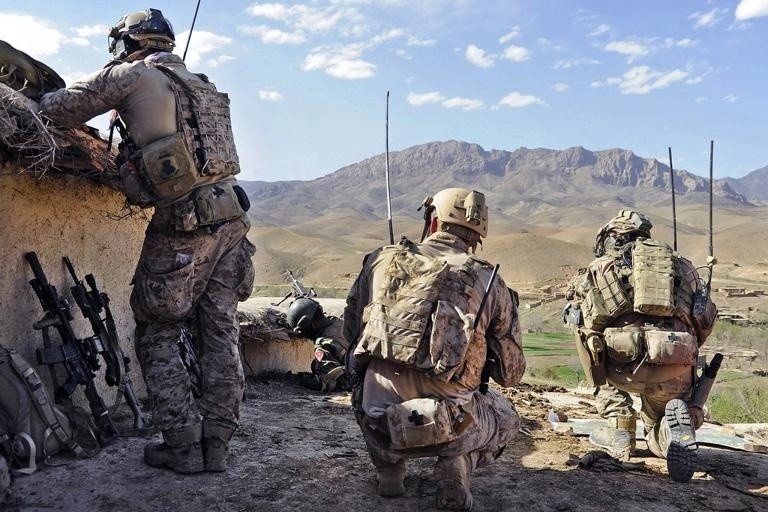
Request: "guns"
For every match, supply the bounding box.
[22,252,119,446]
[60,256,148,430]
[272,271,318,309]
[704,352,724,378]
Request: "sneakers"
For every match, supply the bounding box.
[588,425,638,457]
[375,471,407,497]
[665,398,700,485]
[202,419,236,473]
[432,453,474,511]
[142,426,205,474]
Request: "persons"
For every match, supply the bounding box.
[561,209,719,483]
[286,297,353,391]
[341,186,526,511]
[39,8,258,473]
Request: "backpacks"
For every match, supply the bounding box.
[0,344,88,460]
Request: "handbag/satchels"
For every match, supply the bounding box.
[573,325,698,388]
[193,179,243,227]
[384,397,458,450]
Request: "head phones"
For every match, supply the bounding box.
[592,224,614,258]
[110,33,139,61]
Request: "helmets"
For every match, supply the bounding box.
[285,295,321,335]
[427,186,488,238]
[109,9,178,58]
[606,208,653,239]
